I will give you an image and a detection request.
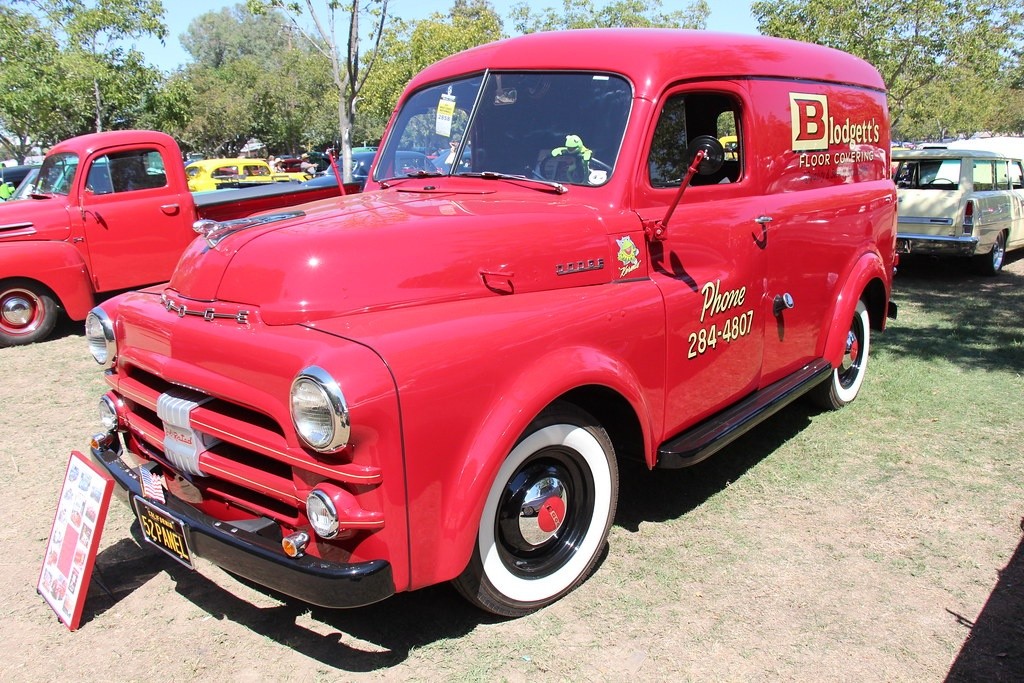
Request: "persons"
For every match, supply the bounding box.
[445,142,463,164]
[274,158,285,172]
[269,155,275,170]
[300,153,318,173]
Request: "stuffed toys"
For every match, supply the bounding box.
[552,135,591,180]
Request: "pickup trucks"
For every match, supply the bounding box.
[0,129,359,344]
[82,28,896,620]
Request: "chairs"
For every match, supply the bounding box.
[110,157,149,193]
[471,95,553,183]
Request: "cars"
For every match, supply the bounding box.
[0,145,471,191]
[888,143,1024,273]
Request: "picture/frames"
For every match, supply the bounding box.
[34,451,114,630]
